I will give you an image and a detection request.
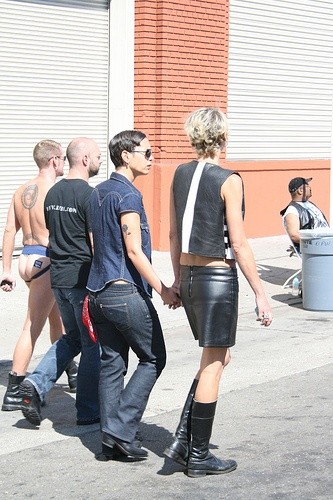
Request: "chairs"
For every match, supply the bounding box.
[281,215,302,289]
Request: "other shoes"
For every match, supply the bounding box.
[76,418,100,425]
[19,380,42,425]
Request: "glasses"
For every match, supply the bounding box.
[48,156,66,161]
[128,149,151,157]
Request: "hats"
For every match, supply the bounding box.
[288,177,312,192]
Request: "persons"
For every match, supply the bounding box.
[0,139,78,411]
[86,129,181,459]
[280,178,329,248]
[164,109,272,476]
[21,136,103,427]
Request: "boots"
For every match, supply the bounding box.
[187,398,237,477]
[1,373,46,410]
[163,379,199,466]
[65,359,80,392]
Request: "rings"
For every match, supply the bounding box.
[263,313,269,318]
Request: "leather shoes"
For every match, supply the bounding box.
[101,431,148,457]
[106,441,143,460]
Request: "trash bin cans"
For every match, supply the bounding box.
[298,227,333,311]
